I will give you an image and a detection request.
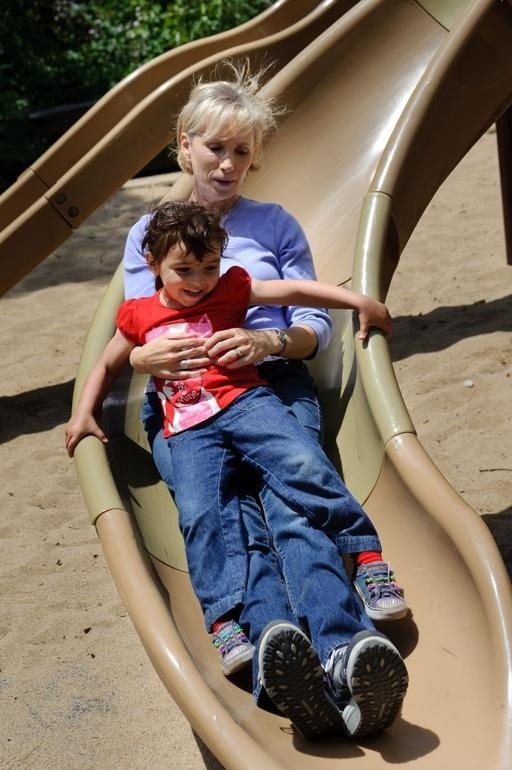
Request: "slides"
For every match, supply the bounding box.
[71,0,512,770]
[0,0,361,297]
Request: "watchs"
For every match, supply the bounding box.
[266,326,287,356]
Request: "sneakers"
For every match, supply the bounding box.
[217,623,257,677]
[353,559,408,622]
[256,619,411,744]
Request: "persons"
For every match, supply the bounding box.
[61,200,413,679]
[120,56,410,745]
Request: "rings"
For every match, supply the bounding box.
[233,348,244,363]
[179,360,188,370]
